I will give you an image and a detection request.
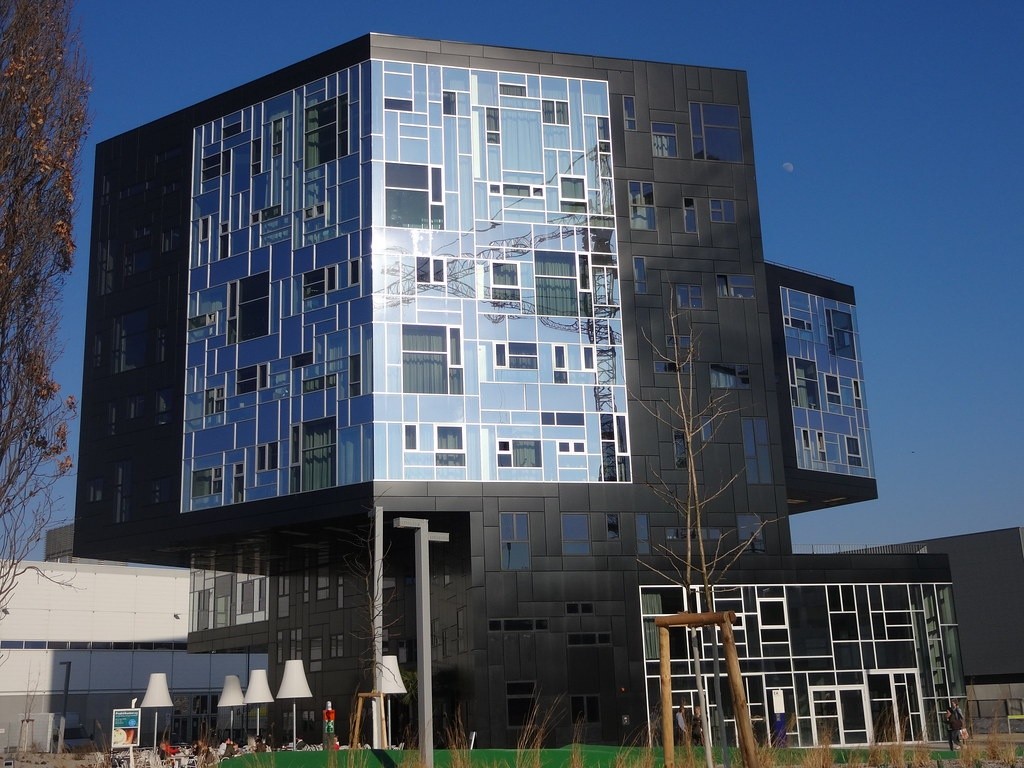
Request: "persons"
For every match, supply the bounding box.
[944,707,963,751]
[693,707,704,746]
[323,701,335,734]
[952,699,964,750]
[651,702,662,746]
[675,705,686,746]
[327,721,334,732]
[158,736,306,761]
[333,736,339,750]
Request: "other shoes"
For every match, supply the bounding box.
[962,745,968,750]
[956,745,960,749]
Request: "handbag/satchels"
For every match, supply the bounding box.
[692,725,701,738]
[951,720,962,730]
[959,728,969,740]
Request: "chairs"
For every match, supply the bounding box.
[113,743,371,768]
